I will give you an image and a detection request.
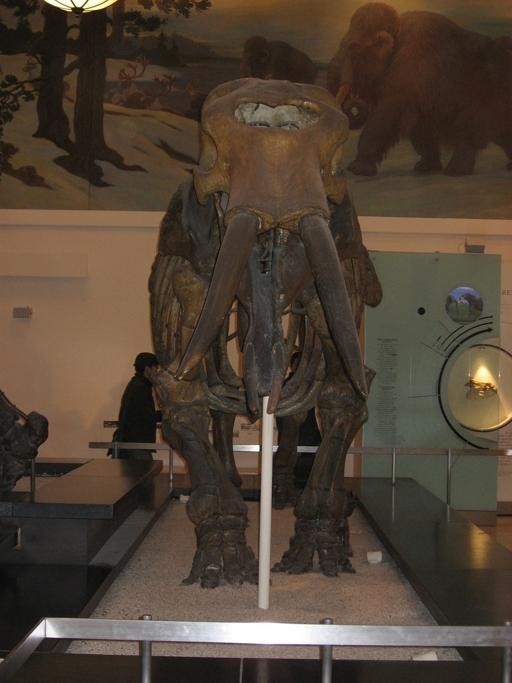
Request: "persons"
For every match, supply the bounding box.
[106,350,162,459]
[274,353,323,474]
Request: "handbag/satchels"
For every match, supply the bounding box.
[107,428,130,457]
